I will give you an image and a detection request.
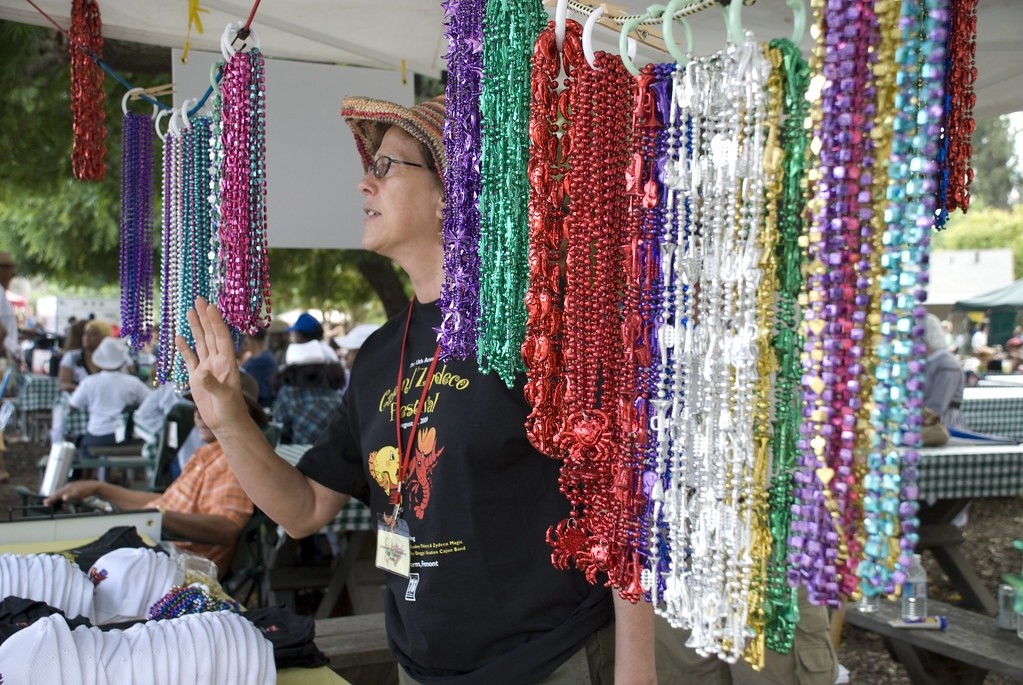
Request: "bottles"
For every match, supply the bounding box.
[901,554,928,623]
[856,579,881,612]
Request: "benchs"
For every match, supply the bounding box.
[304,609,401,685]
[845,596,1023,685]
[913,523,998,615]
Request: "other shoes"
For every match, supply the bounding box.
[833,663,850,684]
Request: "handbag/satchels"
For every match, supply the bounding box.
[238,601,330,669]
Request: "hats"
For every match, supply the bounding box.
[341,94,448,183]
[184,367,267,430]
[92,337,129,372]
[287,314,323,340]
[334,325,378,350]
[269,320,289,334]
[285,342,339,366]
[0,613,277,685]
[1,547,185,626]
[0,252,15,266]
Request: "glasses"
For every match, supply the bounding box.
[368,157,428,178]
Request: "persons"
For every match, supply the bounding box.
[1,248,359,593]
[653,497,856,685]
[175,95,663,685]
[920,311,1023,448]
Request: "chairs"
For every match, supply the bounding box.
[0,369,339,620]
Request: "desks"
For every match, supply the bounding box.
[952,385,1023,442]
[15,374,58,442]
[912,442,1023,500]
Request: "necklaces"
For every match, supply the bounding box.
[440,2,977,675]
[64,0,272,397]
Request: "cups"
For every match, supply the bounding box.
[998,582,1018,630]
